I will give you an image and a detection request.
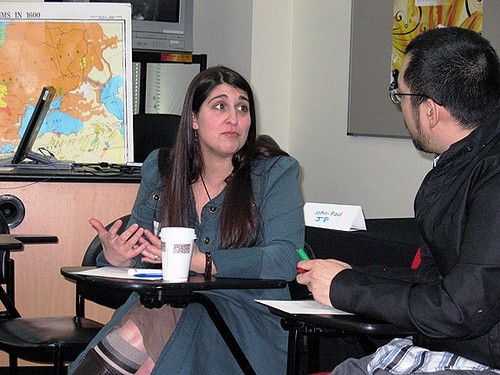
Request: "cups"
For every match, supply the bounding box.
[159,226,196,283]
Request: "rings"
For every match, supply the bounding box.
[155,256,158,261]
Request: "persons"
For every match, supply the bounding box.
[68,65,305,375]
[297,27,500,375]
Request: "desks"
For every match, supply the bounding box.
[0,163,143,367]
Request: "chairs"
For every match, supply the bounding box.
[0,211,423,375]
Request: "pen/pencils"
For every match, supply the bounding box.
[134,274,162,278]
[297,248,311,260]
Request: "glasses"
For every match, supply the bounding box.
[389,87,443,106]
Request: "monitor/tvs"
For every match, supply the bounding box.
[1,86,73,171]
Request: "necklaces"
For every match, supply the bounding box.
[200,173,212,201]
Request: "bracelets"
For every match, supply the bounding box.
[204,252,212,280]
